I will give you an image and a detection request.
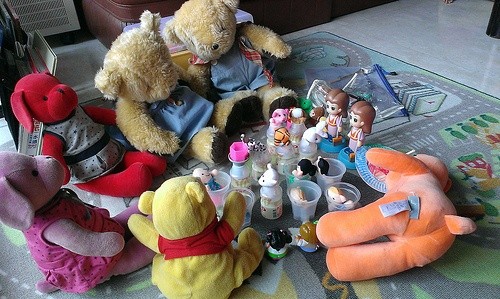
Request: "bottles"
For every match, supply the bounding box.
[290,122,307,154]
[276,146,294,174]
[260,186,283,219]
[251,160,269,181]
[299,137,318,161]
[266,124,284,155]
[229,164,251,190]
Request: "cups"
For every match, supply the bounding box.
[324,182,361,213]
[223,188,255,227]
[205,169,231,218]
[284,164,311,187]
[286,180,322,221]
[314,157,346,193]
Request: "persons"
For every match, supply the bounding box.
[295,222,317,252]
[325,87,349,142]
[346,101,376,153]
[266,229,293,261]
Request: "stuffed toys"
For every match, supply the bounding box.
[269,108,291,129]
[288,107,306,126]
[317,155,329,176]
[127,176,264,299]
[299,127,328,152]
[94,10,243,165]
[316,147,477,281]
[0,152,155,294]
[258,163,280,194]
[274,126,292,148]
[190,168,223,191]
[290,158,354,212]
[164,0,298,121]
[306,107,324,127]
[11,72,165,198]
[240,134,266,152]
